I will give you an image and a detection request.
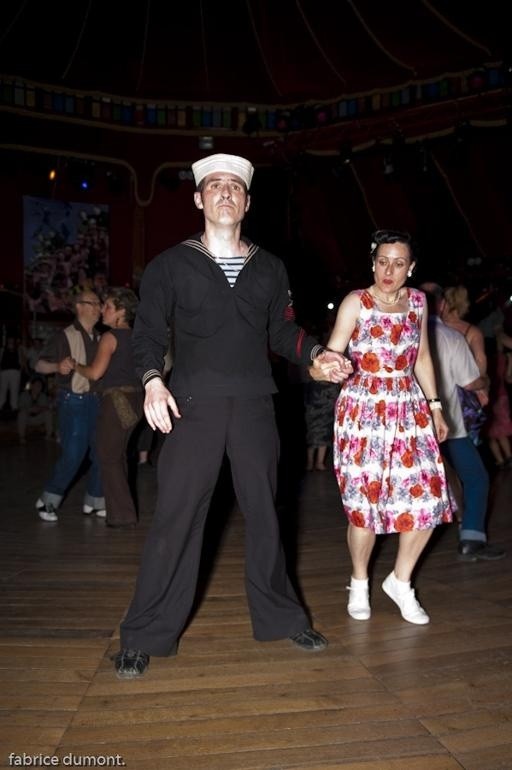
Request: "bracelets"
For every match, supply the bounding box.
[427,399,445,409]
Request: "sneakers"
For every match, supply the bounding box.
[346,574,371,620]
[36,498,58,522]
[83,504,107,517]
[382,572,431,625]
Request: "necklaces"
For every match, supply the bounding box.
[369,285,399,306]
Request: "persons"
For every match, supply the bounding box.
[415,280,504,565]
[0,336,59,445]
[32,289,107,522]
[31,206,109,312]
[309,225,450,625]
[480,302,511,468]
[439,288,490,536]
[74,286,144,531]
[132,418,158,471]
[296,378,340,473]
[113,153,355,677]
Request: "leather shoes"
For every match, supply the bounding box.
[459,539,507,560]
[115,648,149,678]
[290,629,327,649]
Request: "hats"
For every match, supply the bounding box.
[192,152,255,191]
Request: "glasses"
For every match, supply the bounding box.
[79,300,101,306]
